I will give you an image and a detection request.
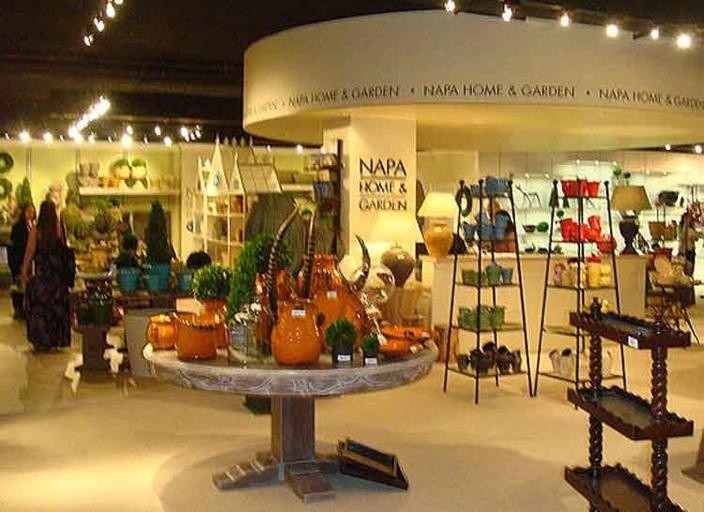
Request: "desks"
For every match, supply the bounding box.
[147,344,432,501]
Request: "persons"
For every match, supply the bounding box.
[674,212,699,277]
[5,201,38,320]
[18,200,77,355]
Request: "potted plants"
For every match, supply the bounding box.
[112,200,193,298]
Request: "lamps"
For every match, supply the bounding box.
[610,184,652,257]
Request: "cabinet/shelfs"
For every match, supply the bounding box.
[442,176,533,406]
[565,296,697,512]
[534,176,630,399]
[191,131,280,270]
